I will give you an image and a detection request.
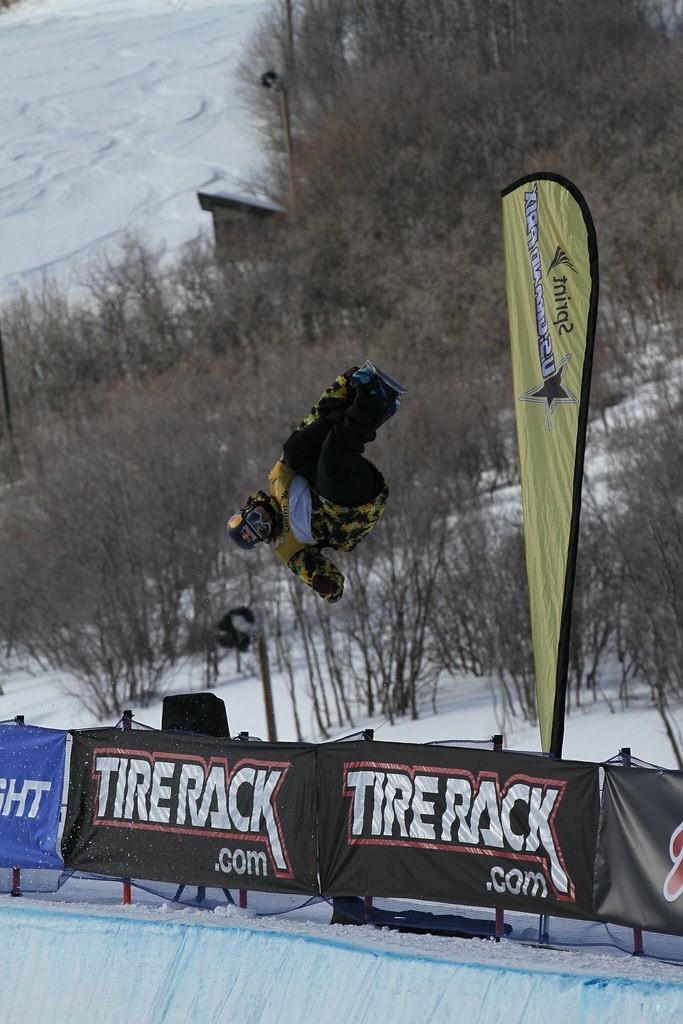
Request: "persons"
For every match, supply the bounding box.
[226,361,408,604]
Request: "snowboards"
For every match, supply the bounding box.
[362,361,410,395]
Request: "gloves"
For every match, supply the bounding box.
[311,574,338,595]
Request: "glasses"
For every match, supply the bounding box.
[246,511,272,541]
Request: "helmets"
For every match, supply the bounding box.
[227,510,261,551]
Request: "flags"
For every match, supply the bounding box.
[498,170,600,757]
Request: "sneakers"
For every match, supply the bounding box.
[352,373,389,397]
